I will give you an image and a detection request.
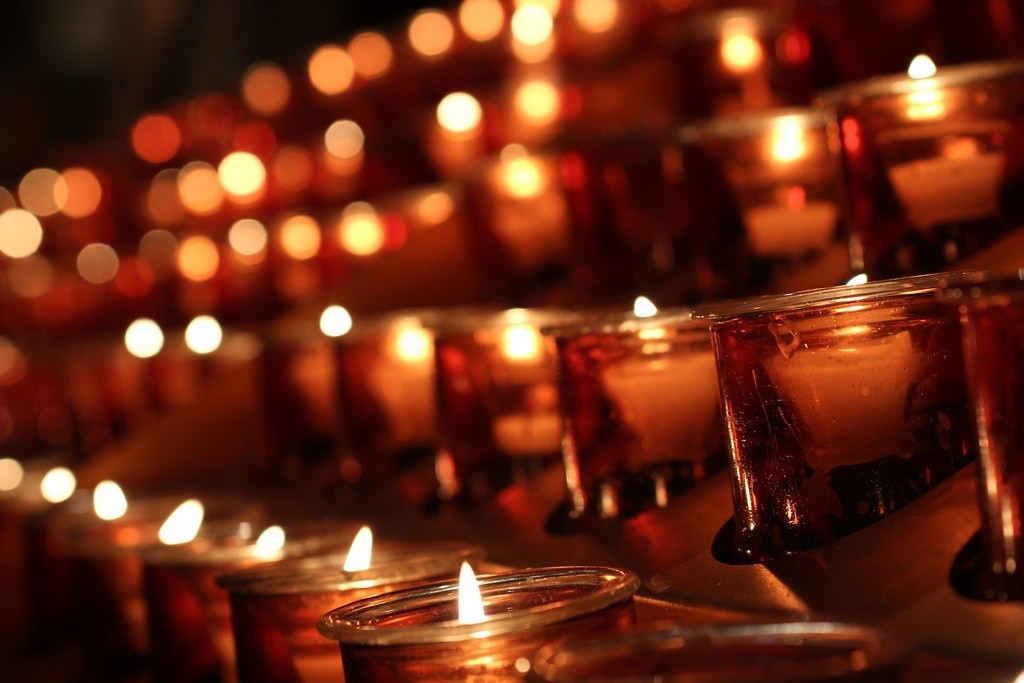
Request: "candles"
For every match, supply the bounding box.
[5,0,1023,681]
